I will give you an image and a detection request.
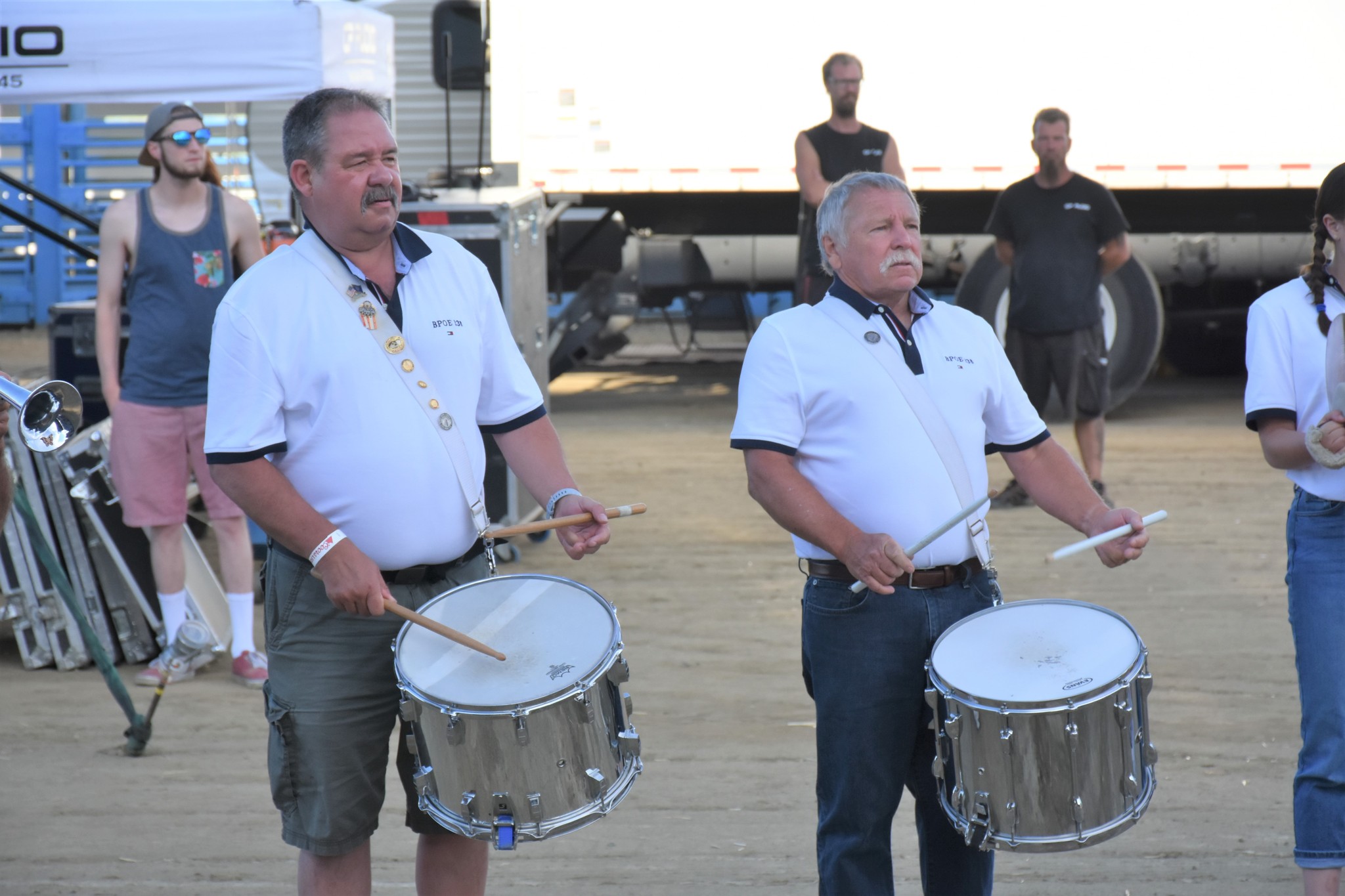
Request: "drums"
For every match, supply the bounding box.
[390,572,644,851]
[924,598,1159,853]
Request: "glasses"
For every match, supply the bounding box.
[160,129,210,145]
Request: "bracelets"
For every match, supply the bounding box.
[309,529,347,567]
[545,488,582,520]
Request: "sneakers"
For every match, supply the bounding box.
[231,650,267,690]
[136,661,196,685]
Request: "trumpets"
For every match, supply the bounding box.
[0,376,84,453]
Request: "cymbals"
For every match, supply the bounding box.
[1324,312,1345,429]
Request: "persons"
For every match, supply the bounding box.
[730,169,1149,896]
[0,370,16,534]
[96,102,268,689]
[204,87,616,896]
[984,109,1131,509]
[792,53,906,306]
[1244,163,1345,896]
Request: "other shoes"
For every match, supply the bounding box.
[991,481,1030,511]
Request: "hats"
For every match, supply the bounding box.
[138,102,203,166]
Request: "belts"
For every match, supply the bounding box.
[381,538,483,585]
[803,560,984,586]
